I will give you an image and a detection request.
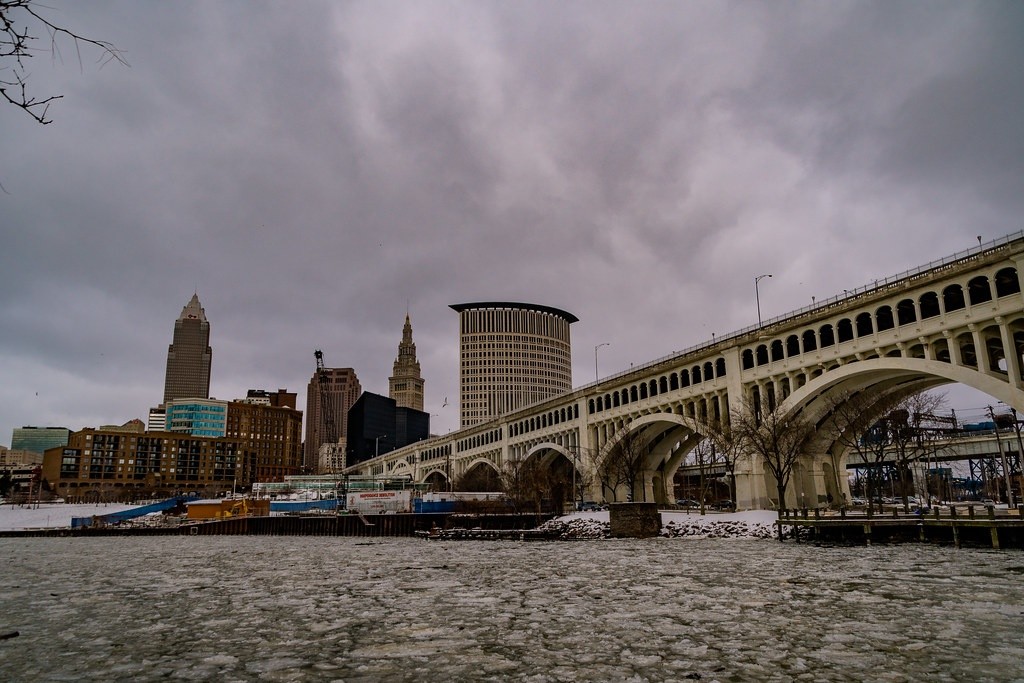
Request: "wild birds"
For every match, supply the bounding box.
[429,410,442,418]
[442,397,449,408]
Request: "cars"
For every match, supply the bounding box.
[984,499,995,508]
[882,497,894,504]
[894,497,903,504]
[851,498,865,505]
[578,500,602,511]
[867,497,882,503]
[721,500,736,508]
[677,500,700,506]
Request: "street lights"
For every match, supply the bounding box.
[376,435,386,457]
[755,275,772,330]
[594,343,609,386]
